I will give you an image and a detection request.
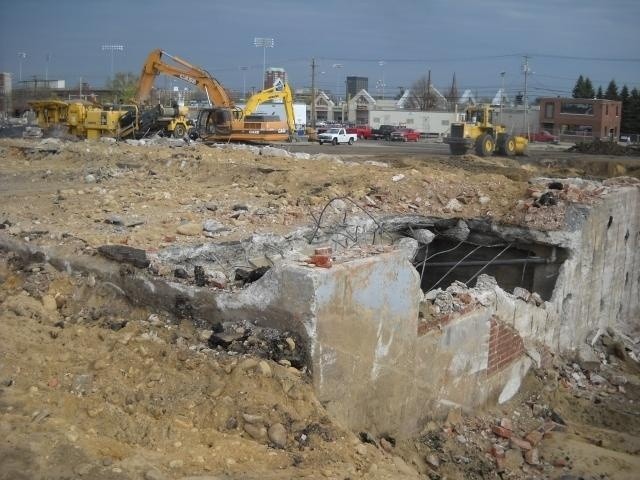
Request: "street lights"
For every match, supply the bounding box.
[102,42,126,87]
[253,35,275,92]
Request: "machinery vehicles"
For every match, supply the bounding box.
[446,103,527,158]
[31,56,300,145]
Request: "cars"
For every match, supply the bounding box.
[391,128,421,142]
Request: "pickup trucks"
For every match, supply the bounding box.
[319,128,359,145]
[347,125,371,139]
[372,125,396,139]
